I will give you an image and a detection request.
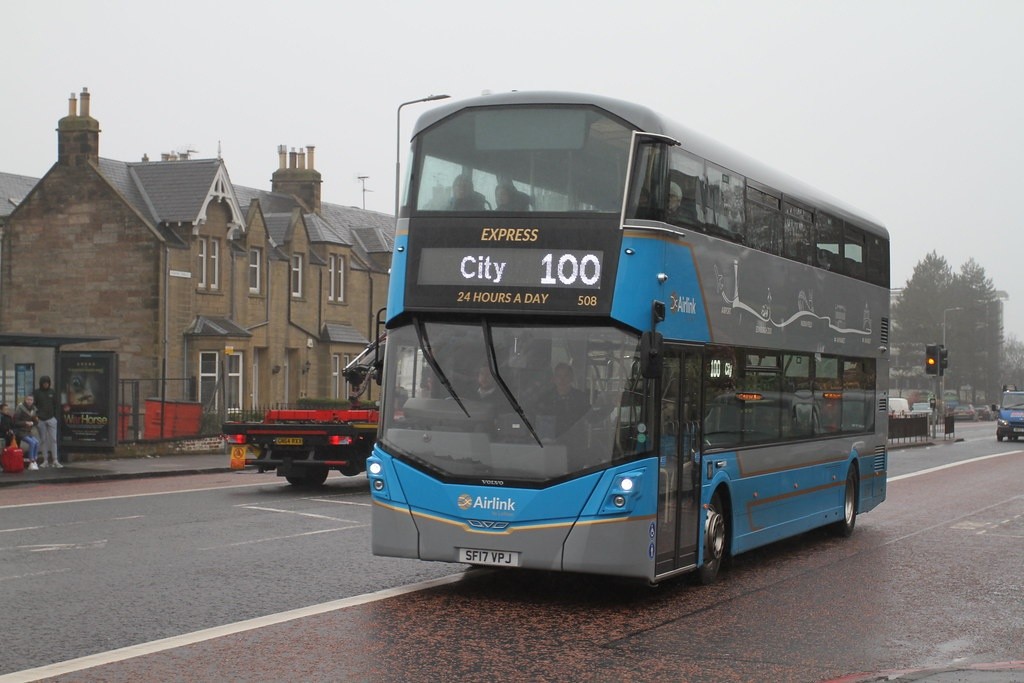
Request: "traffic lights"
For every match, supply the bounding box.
[926,344,937,375]
[938,343,948,377]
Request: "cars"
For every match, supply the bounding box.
[996,391,1024,441]
[888,398,997,421]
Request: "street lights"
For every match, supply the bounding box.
[395,94,450,217]
[941,307,963,398]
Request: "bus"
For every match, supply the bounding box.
[379,89,889,577]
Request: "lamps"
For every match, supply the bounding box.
[302,361,310,374]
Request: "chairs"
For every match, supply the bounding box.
[661,167,865,281]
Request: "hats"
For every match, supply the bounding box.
[656,181,682,198]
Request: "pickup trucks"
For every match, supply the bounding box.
[219,403,381,486]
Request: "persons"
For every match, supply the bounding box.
[0,375,64,471]
[466,362,510,408]
[655,181,705,234]
[446,173,484,211]
[544,360,587,412]
[494,184,525,212]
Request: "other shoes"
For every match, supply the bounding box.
[51,462,64,469]
[39,462,50,468]
[27,462,39,471]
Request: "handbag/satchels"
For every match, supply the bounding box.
[2,434,24,473]
